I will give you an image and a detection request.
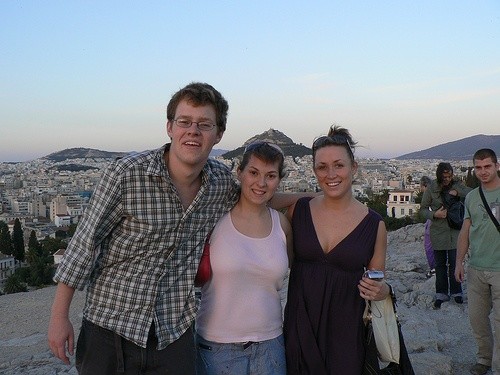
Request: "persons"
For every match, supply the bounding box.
[194,141,290,375]
[48,82,324,375]
[420,177,437,277]
[284,124,391,375]
[454,148,500,375]
[420,162,473,310]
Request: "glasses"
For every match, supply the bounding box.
[170,117,219,131]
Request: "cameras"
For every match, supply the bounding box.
[364,270,384,280]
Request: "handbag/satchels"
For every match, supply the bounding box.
[445,190,465,230]
[193,224,216,287]
[370,299,400,365]
[364,320,416,375]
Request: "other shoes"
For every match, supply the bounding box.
[454,295,464,304]
[470,361,491,375]
[435,298,443,308]
